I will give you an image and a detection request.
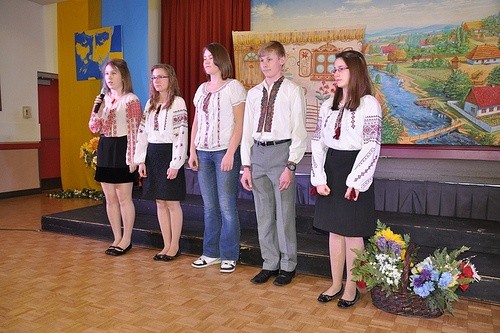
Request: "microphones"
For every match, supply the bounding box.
[94,88,108,113]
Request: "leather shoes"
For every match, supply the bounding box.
[153,249,181,262]
[273,269,296,285]
[250,269,279,284]
[104,243,132,256]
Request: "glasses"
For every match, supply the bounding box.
[331,67,349,75]
[151,75,168,80]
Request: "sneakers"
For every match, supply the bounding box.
[191,254,221,268]
[220,260,236,273]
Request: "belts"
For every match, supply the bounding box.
[254,139,291,146]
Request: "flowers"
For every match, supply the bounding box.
[80,136,100,170]
[349,218,480,315]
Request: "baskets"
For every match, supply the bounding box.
[370,242,445,319]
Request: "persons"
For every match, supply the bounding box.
[89,57,143,258]
[132,63,189,262]
[187,42,247,274]
[310,51,383,309]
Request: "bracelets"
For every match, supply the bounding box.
[241,165,251,171]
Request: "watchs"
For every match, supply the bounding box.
[239,40,308,287]
[285,161,297,172]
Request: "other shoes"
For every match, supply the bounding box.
[337,287,361,307]
[317,283,344,302]
